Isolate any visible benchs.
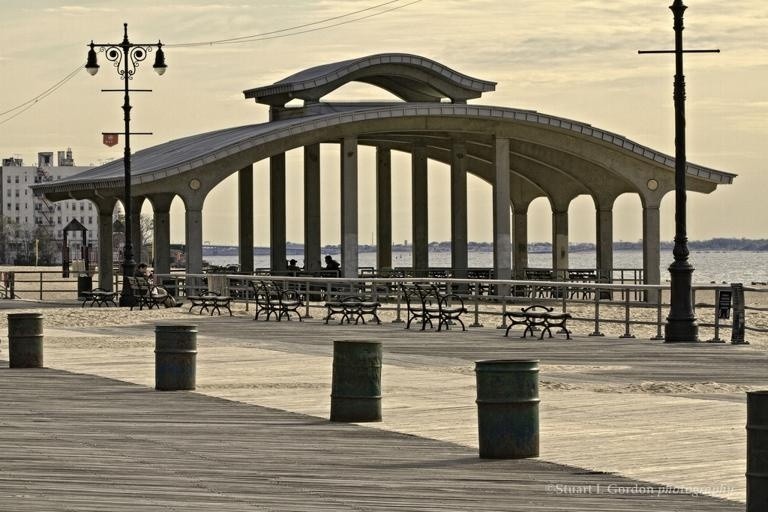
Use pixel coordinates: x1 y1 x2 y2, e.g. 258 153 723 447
322 285 572 342
81 280 303 325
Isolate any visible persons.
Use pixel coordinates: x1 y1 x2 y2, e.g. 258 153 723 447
287 259 301 276
323 255 341 277
134 262 183 308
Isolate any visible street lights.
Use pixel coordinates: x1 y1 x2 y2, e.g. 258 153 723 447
84 21 171 306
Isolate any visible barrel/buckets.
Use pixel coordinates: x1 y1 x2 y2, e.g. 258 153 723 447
474 358 540 460
330 340 383 422
78 277 92 299
746 390 768 512
7 313 43 367
154 325 198 391
